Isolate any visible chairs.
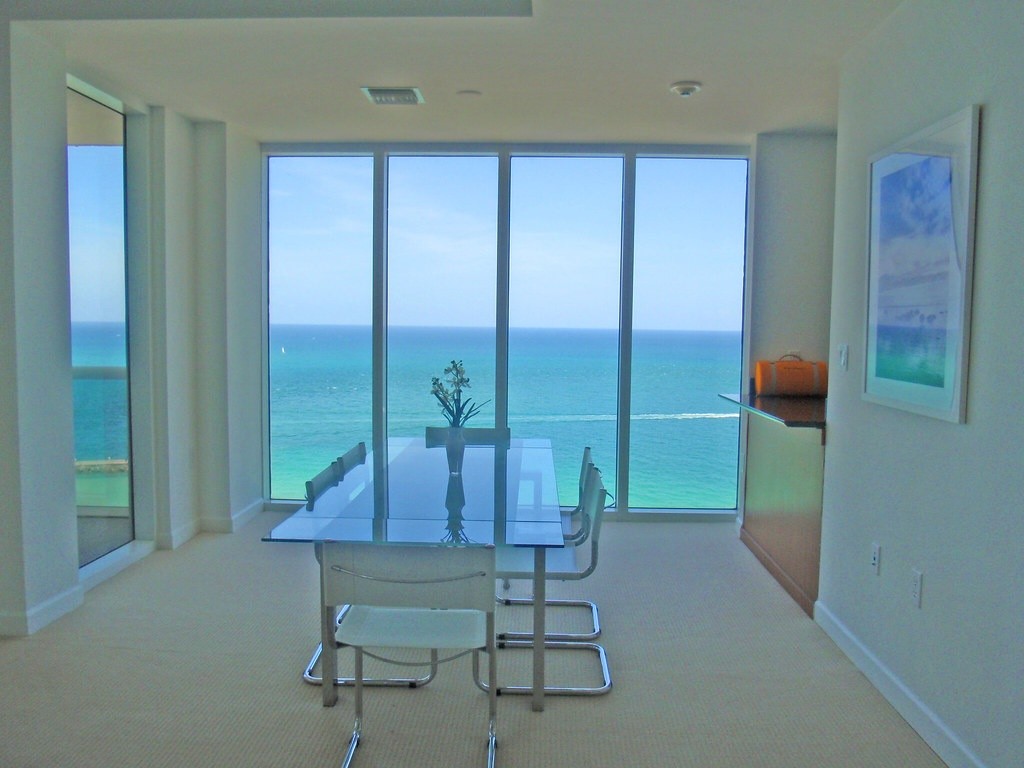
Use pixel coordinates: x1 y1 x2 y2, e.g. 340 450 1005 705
304 440 614 768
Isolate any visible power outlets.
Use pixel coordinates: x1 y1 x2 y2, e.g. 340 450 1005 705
907 568 922 609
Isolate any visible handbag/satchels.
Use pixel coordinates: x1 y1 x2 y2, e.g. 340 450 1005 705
755 354 828 396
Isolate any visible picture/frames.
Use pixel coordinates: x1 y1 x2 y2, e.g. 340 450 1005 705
861 104 983 426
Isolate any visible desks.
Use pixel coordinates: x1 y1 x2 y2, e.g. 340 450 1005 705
263 437 564 714
719 391 826 620
